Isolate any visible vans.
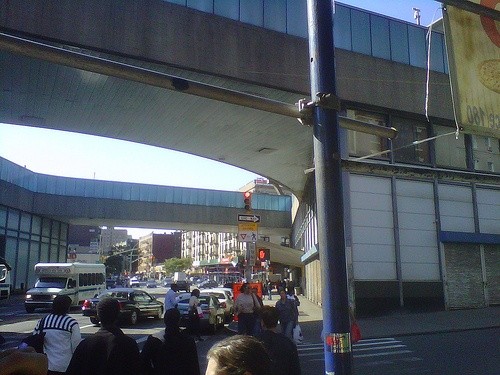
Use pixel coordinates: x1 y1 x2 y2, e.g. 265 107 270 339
25 263 107 313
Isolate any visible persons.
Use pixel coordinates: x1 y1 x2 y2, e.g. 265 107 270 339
276 286 300 338
221 277 294 300
188 289 204 340
258 305 300 375
165 284 180 310
152 308 201 375
234 283 263 335
206 335 274 375
33 295 81 375
66 297 147 375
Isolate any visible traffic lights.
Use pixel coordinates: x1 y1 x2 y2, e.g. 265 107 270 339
259 249 271 261
243 191 251 211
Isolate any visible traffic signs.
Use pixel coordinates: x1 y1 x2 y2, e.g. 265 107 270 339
237 214 261 224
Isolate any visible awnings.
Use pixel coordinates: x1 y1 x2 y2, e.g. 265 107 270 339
440 231 500 244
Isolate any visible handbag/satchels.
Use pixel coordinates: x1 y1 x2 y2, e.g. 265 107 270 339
293 324 304 344
189 304 201 322
18 315 47 353
251 293 262 315
164 303 186 325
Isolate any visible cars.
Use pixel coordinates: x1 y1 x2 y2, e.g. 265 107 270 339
83 288 164 326
107 271 234 329
